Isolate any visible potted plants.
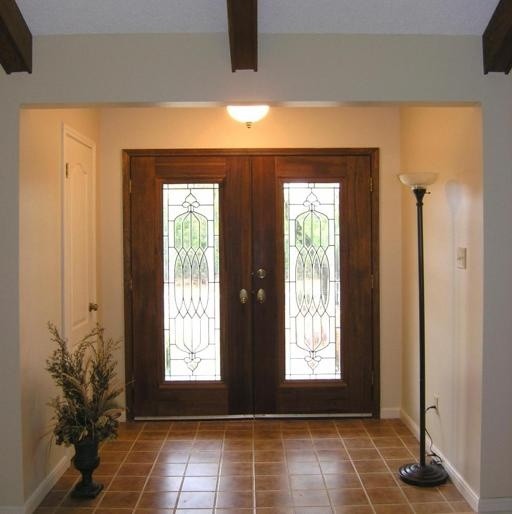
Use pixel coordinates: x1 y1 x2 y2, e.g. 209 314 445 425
46 321 131 498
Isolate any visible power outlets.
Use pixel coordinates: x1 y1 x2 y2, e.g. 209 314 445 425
433 393 439 416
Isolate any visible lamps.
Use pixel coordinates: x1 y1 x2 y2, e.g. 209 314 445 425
398 170 449 487
227 105 269 129
